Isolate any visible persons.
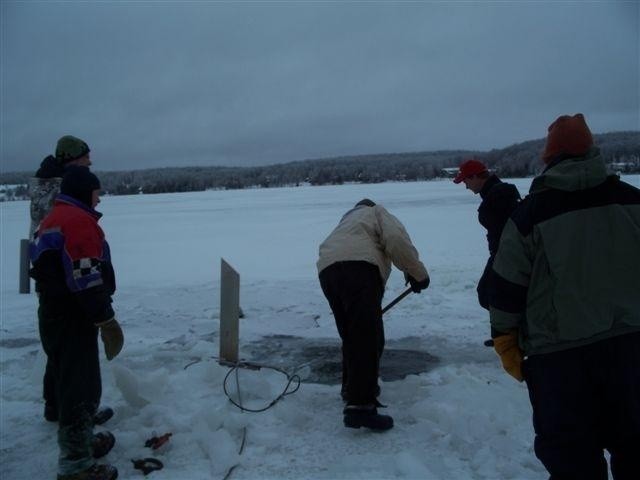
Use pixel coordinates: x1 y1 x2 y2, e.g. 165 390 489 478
26 165 126 480
317 198 431 434
29 134 113 426
483 111 639 480
451 154 523 349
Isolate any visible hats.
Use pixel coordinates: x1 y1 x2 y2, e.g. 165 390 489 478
453 160 488 184
55 135 90 163
542 114 593 163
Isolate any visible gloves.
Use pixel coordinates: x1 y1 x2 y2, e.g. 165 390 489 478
492 331 524 383
405 273 429 294
100 319 125 361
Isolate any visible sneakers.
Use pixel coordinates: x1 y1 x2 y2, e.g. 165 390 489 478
42 405 118 480
343 405 394 431
